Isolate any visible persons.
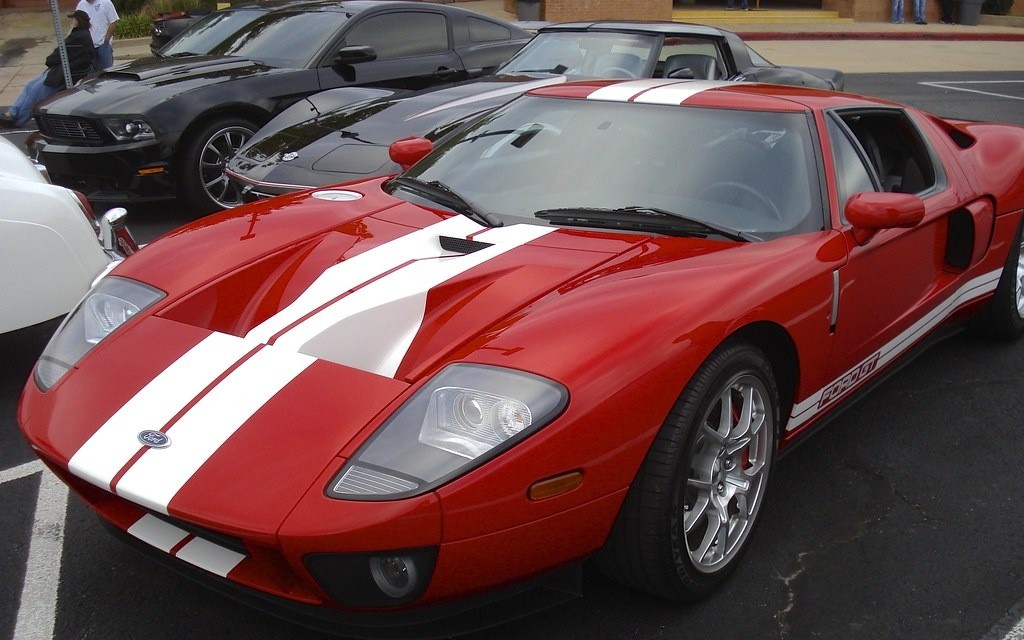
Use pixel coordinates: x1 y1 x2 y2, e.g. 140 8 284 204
891 0 927 24
0 10 96 129
76 0 120 76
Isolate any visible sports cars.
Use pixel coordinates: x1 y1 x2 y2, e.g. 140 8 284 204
226 17 845 219
17 76 1024 640
24 0 538 224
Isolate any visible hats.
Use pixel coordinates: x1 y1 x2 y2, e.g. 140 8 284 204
67 10 90 21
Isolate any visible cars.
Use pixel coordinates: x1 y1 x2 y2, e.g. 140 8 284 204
149 6 211 58
0 135 148 362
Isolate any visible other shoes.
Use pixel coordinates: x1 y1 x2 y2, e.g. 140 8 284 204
0 112 16 128
